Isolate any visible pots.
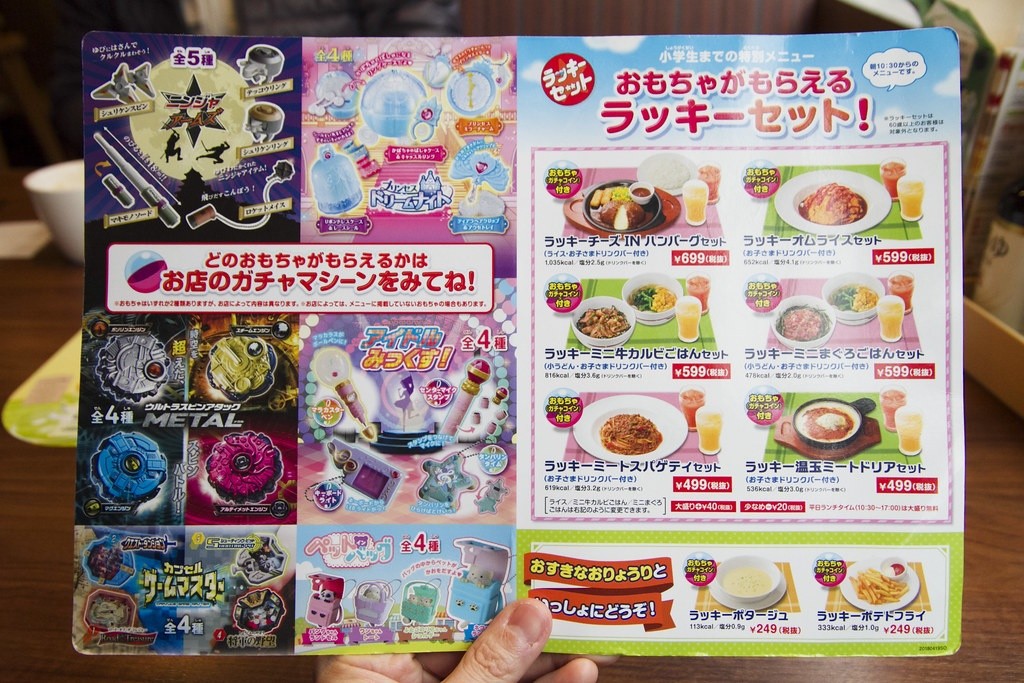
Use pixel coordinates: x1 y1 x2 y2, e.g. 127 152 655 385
792 397 876 449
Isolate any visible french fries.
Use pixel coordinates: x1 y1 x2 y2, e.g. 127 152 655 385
847 568 909 604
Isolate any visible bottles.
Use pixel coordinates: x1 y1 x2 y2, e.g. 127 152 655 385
970 178 1024 333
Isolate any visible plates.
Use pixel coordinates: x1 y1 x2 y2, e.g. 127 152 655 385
774 170 893 235
708 572 787 611
572 394 689 464
840 556 920 612
582 179 662 233
637 153 697 196
1 325 82 448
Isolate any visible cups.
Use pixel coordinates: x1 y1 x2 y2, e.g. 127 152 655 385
675 296 702 343
879 388 907 433
888 270 915 315
22 158 84 266
697 165 721 205
894 406 922 456
896 175 925 222
879 159 906 202
695 405 723 456
683 179 709 226
686 275 711 315
679 388 705 432
876 295 905 343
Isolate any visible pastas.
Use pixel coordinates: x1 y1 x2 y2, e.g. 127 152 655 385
602 414 657 454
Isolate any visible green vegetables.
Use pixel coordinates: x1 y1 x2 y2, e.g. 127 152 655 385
830 287 856 312
633 287 657 311
776 304 831 340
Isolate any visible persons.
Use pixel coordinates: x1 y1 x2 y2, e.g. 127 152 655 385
315 598 619 683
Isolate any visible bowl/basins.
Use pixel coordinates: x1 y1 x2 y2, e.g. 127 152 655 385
629 182 654 204
879 557 909 580
715 555 781 603
822 271 886 326
570 296 636 351
621 271 684 325
770 295 837 351
